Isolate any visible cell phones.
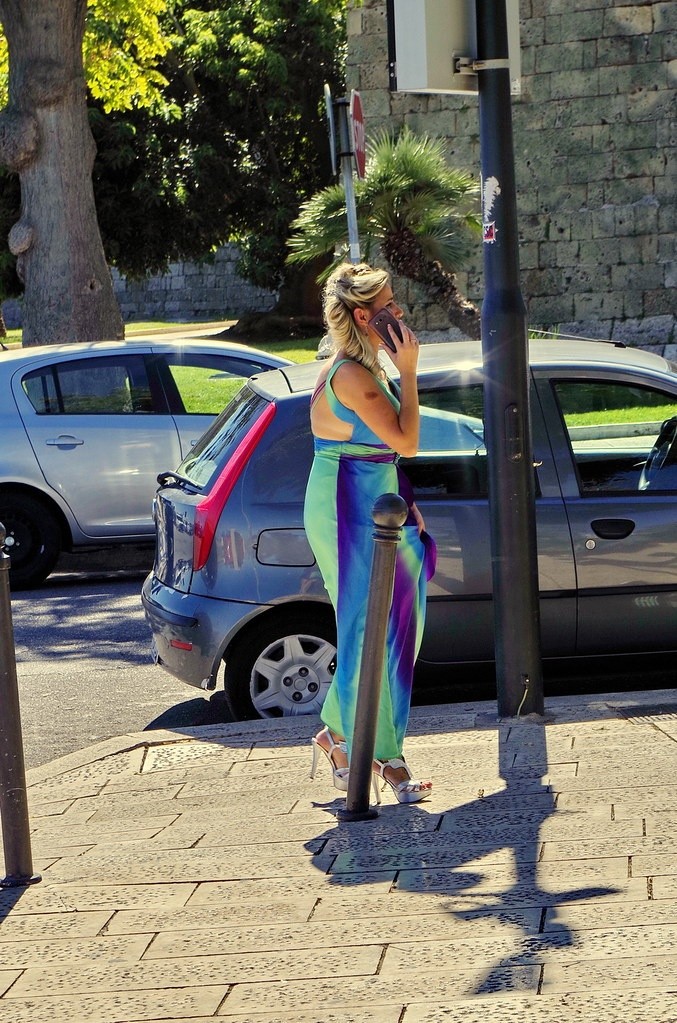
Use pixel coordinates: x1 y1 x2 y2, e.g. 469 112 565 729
368 308 404 353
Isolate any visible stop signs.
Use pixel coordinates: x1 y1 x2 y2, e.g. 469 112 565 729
346 89 367 183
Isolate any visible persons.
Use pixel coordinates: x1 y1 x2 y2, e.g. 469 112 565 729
304 265 437 806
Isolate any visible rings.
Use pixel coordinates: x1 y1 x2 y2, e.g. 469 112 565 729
410 339 417 342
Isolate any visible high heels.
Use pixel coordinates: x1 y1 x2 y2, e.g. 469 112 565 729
372 754 432 803
310 726 348 792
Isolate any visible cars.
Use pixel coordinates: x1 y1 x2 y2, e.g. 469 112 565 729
139 332 676 718
0 337 297 589
